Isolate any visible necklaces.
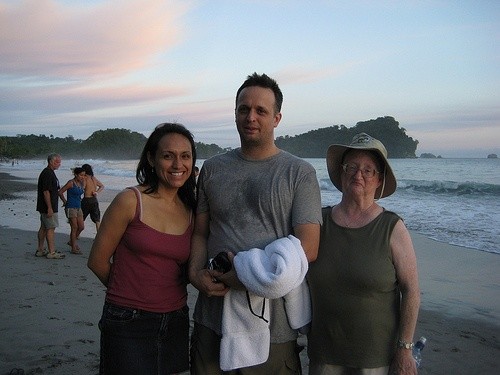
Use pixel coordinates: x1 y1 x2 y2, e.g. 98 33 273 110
340 202 375 229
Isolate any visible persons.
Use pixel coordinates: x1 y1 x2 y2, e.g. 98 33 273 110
305 132 421 375
59 167 84 254
194 166 199 178
35 153 67 258
81 164 104 231
188 72 322 375
87 122 195 375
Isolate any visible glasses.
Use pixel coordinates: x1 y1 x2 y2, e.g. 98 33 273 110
345 163 376 178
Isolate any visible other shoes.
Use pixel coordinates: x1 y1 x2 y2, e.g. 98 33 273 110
34 249 65 259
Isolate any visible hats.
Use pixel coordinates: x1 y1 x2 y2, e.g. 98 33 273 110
326 133 397 201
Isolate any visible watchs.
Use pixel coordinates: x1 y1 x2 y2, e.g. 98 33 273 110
398 339 414 350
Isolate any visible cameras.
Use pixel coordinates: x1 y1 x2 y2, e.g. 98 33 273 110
204 251 233 284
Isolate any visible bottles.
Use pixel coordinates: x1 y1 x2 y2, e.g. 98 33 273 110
388 336 427 375
